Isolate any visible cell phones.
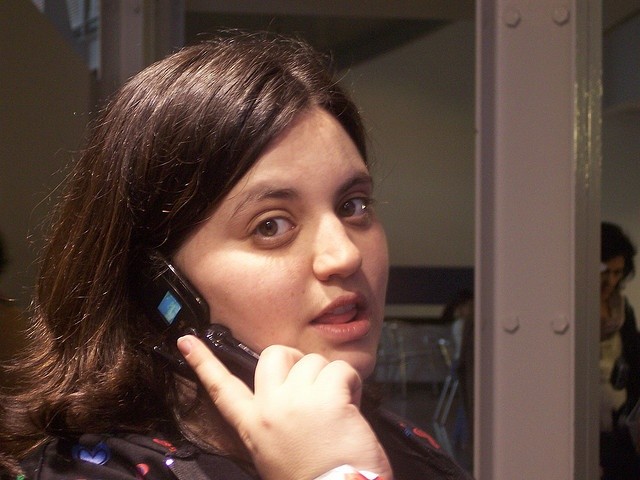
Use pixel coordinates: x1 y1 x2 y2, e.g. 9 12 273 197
132 253 260 394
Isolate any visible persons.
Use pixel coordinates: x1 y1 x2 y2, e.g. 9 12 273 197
0 28 477 480
599 221 640 480
